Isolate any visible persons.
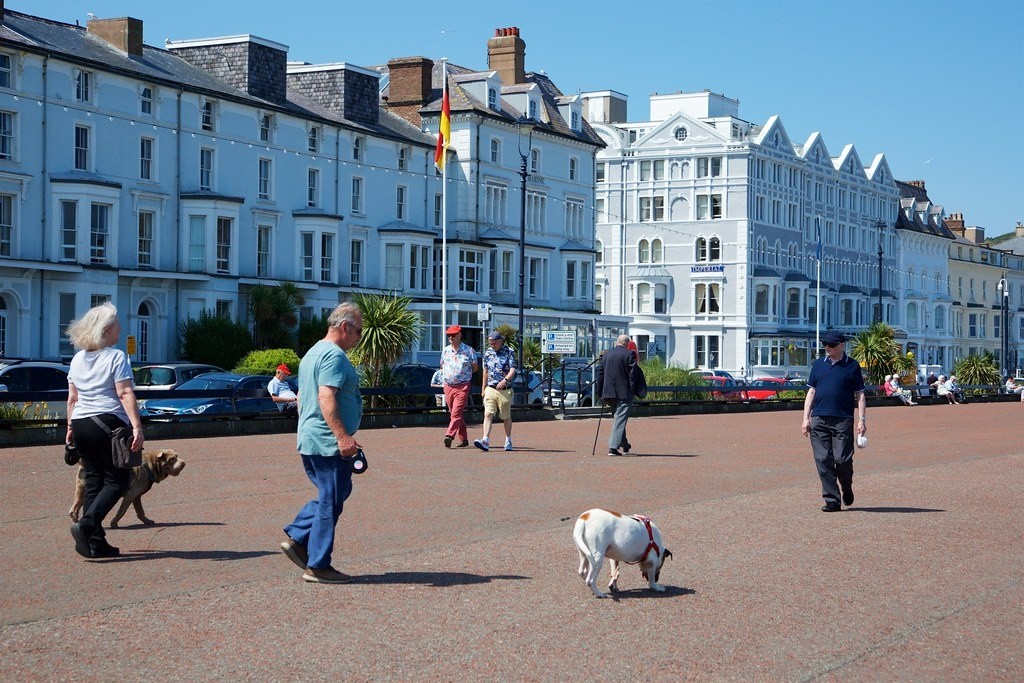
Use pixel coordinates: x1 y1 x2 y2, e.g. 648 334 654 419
66 302 144 558
431 365 443 406
927 372 938 398
267 365 297 413
945 376 968 404
916 374 922 396
884 373 918 405
890 374 912 405
801 330 867 511
930 375 959 405
281 302 364 584
441 325 477 447
1006 377 1024 402
596 334 637 456
474 331 517 451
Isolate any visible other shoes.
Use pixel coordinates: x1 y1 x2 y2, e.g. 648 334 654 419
623 444 631 452
821 501 841 512
474 439 489 451
608 448 622 456
456 440 468 447
842 486 854 506
505 441 512 450
280 538 309 569
303 564 352 583
69 522 92 557
89 538 120 557
444 437 452 447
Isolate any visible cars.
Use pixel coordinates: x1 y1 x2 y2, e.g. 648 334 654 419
0 357 70 429
138 369 299 424
133 363 229 403
509 360 600 409
356 362 436 408
689 370 809 404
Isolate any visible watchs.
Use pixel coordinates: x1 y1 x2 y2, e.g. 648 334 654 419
858 416 866 421
504 378 509 382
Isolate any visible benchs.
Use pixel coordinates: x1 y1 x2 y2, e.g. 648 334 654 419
628 385 1021 417
0 383 544 448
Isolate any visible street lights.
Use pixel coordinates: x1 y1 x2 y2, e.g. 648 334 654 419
513 109 541 370
997 277 1009 384
869 217 889 323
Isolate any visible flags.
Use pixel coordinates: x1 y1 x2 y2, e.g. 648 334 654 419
434 76 450 174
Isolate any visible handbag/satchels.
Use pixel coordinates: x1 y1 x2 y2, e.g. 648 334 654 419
111 425 143 467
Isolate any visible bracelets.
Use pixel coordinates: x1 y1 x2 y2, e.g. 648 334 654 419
133 428 143 432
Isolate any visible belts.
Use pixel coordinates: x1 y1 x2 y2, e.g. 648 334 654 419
489 386 512 390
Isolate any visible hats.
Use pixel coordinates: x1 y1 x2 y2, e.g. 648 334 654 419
820 330 845 344
488 331 506 343
893 374 900 378
885 375 891 381
277 365 291 374
951 376 956 379
446 325 461 335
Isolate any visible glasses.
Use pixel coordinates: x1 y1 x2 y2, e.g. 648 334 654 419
821 341 841 349
447 334 456 338
344 323 363 335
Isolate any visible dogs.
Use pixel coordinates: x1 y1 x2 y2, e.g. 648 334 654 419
67 447 188 531
570 505 676 600
